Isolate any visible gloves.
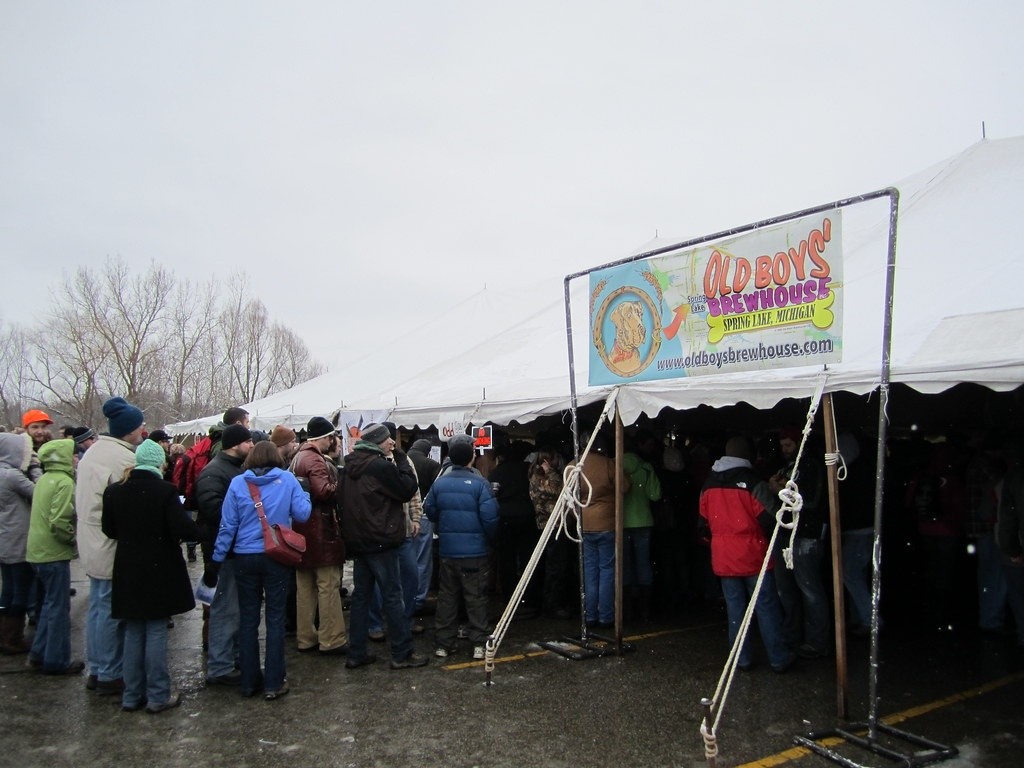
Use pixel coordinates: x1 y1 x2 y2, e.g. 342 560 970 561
203 560 221 588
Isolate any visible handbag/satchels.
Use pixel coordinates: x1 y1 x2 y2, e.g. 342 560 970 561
263 523 306 565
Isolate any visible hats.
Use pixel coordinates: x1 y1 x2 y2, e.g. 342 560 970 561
778 425 803 440
103 398 143 438
448 435 477 448
360 422 390 444
135 430 187 467
21 409 54 428
222 417 335 450
712 456 751 472
73 426 94 444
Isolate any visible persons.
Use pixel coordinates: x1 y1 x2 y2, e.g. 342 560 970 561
1 397 1023 712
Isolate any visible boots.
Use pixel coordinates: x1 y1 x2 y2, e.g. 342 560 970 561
0 607 32 654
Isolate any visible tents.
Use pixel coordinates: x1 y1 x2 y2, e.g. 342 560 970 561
162 134 1024 757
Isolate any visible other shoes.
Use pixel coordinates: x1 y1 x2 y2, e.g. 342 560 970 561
410 617 425 633
587 619 614 630
369 628 385 642
742 644 817 673
299 640 351 656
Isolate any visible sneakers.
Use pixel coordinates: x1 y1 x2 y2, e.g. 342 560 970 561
436 624 491 657
392 652 429 668
87 675 125 696
123 692 179 712
28 656 85 675
345 654 376 668
207 669 289 700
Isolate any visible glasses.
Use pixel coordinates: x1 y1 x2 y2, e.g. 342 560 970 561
328 435 333 441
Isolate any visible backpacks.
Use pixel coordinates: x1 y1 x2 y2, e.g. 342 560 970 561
174 433 213 499
